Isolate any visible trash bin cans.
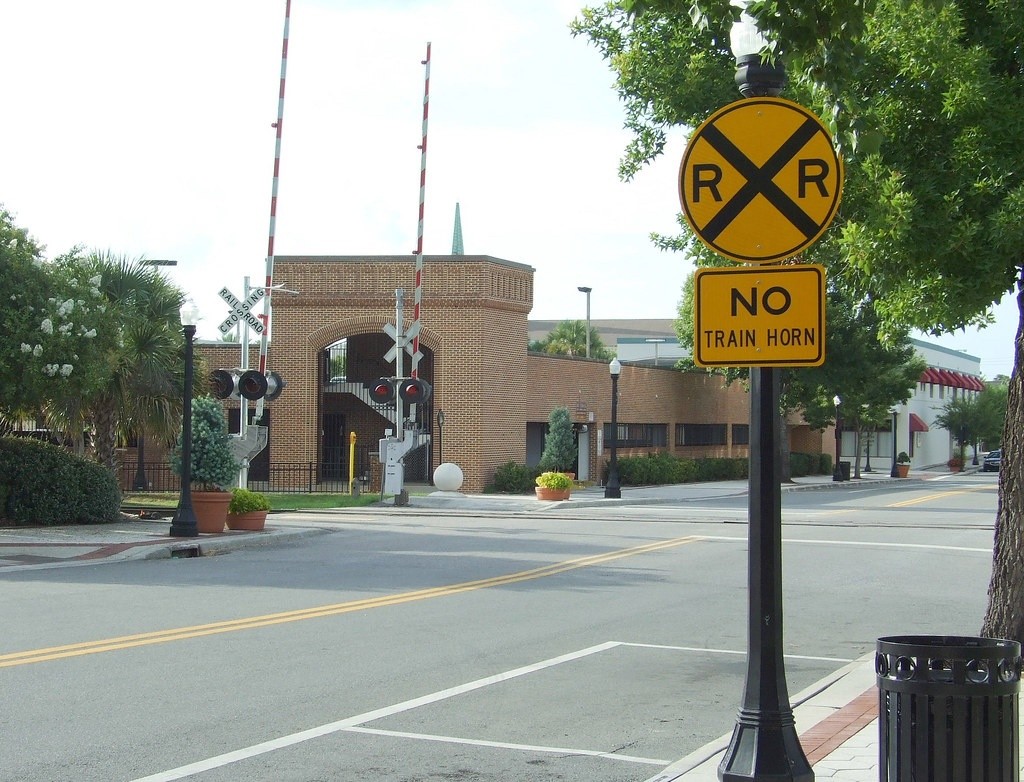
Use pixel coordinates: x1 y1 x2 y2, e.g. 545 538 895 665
874 635 1021 782
839 461 851 481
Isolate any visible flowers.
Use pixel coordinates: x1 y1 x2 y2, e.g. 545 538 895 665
947 458 961 467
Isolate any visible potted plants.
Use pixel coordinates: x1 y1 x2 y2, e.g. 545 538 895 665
173 393 249 533
540 405 576 500
896 452 911 478
535 472 574 501
225 487 270 530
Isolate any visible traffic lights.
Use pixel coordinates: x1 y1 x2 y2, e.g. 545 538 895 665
239 371 268 400
399 379 423 403
370 379 393 404
208 370 234 400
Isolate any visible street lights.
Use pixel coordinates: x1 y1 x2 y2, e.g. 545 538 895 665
168 299 199 537
833 395 844 482
578 287 592 357
891 402 900 478
604 357 621 498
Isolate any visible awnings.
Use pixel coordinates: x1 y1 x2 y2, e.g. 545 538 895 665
916 366 987 391
909 413 929 432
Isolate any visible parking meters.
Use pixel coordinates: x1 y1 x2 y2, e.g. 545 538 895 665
350 431 356 494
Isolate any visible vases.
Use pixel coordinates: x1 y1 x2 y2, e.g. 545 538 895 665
949 467 960 471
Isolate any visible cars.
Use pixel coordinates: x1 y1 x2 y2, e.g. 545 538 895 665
983 450 1000 472
